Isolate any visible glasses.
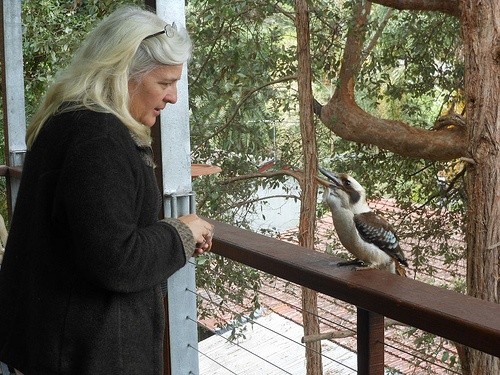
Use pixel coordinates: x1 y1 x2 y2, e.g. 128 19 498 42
143 21 177 41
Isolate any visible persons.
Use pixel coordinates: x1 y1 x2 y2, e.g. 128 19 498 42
1 5 214 375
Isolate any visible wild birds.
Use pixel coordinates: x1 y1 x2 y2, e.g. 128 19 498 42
313 167 409 279
